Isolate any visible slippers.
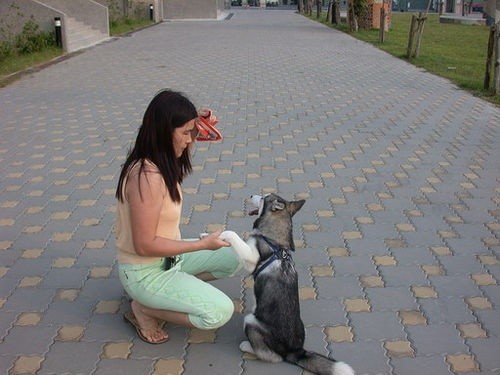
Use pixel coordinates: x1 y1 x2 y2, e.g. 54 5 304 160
124 309 169 343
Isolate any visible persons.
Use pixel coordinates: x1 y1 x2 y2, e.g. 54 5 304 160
114 89 247 344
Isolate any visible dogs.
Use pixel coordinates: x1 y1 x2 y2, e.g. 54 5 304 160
199 193 355 374
